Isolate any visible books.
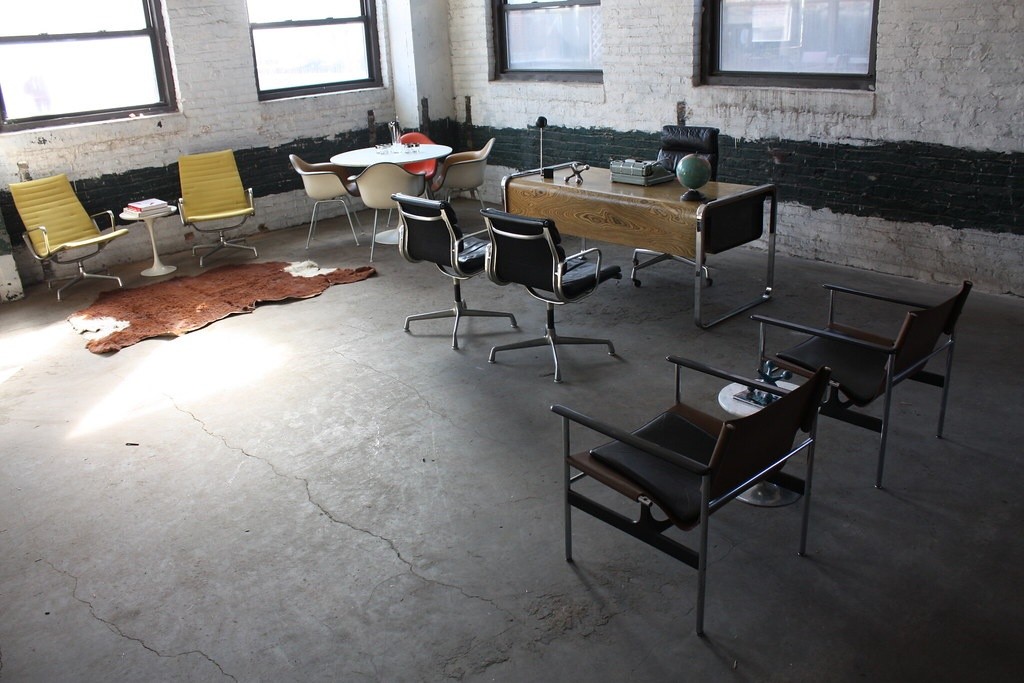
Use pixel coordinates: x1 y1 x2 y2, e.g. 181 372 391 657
123 207 171 217
127 197 168 212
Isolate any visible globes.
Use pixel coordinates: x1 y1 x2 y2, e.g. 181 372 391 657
676 151 712 201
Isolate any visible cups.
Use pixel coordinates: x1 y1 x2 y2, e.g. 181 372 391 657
404 142 419 153
375 143 391 154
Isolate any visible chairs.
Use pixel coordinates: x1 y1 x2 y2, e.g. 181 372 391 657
8 173 130 303
289 154 365 250
549 354 832 638
346 161 429 262
750 280 973 490
479 207 622 384
430 137 495 209
630 124 720 289
177 148 258 268
390 192 517 351
386 132 438 228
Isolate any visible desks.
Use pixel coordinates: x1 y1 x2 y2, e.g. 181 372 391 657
501 160 778 330
717 379 805 509
329 144 453 245
119 205 177 277
